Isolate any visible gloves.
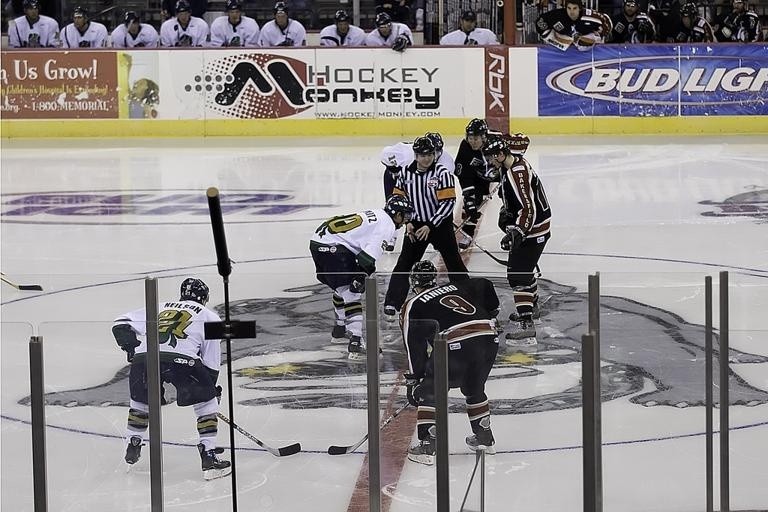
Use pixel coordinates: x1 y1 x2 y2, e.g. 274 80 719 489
403 373 426 407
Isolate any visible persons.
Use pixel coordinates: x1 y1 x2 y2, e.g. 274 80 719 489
0 0 501 55
309 193 414 356
534 0 767 54
112 278 231 471
381 119 552 341
399 260 498 456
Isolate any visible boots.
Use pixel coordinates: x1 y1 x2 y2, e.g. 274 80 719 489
197 443 230 471
332 305 540 355
408 423 437 456
466 414 495 446
125 437 145 464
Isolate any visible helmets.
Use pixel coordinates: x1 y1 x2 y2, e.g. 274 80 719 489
181 278 209 306
384 119 508 289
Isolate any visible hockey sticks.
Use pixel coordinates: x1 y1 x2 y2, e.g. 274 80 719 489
453 223 508 266
0 276 41 291
216 412 301 456
329 402 410 454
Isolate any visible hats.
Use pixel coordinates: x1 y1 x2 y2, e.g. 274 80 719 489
461 11 476 20
